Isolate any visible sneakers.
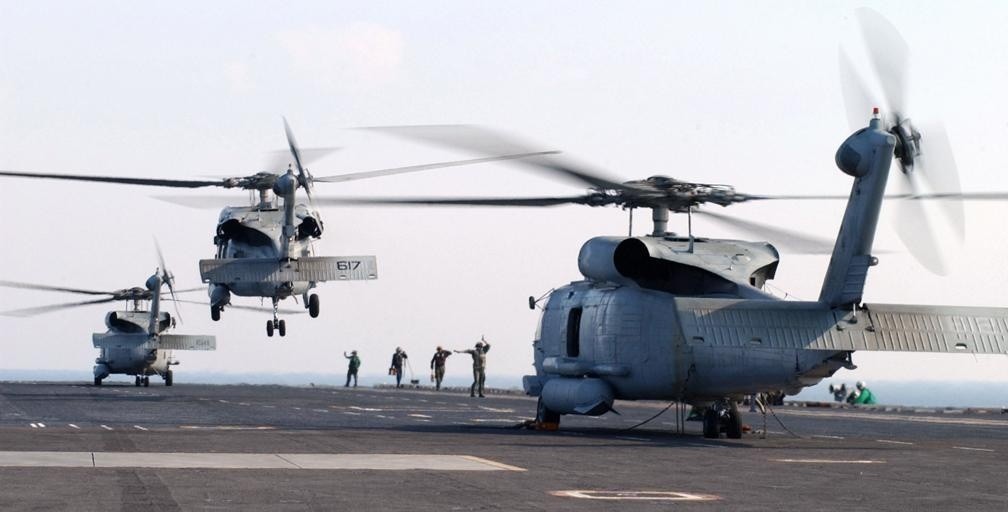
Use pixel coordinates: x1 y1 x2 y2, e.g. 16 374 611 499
470 394 476 397
478 394 486 398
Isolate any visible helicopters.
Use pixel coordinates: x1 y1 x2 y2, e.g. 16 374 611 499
0 118 574 337
367 5 1006 439
1 270 306 390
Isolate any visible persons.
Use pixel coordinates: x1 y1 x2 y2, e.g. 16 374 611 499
343 350 361 388
390 346 408 389
453 335 491 399
850 380 878 404
846 389 860 403
429 346 452 391
